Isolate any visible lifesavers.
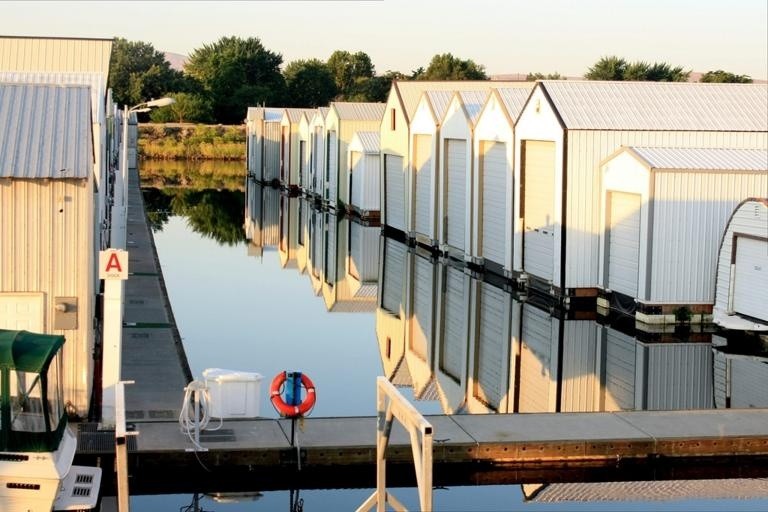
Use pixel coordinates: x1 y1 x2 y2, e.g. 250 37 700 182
269 370 316 415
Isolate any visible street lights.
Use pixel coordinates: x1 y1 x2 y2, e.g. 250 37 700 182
121 95 175 210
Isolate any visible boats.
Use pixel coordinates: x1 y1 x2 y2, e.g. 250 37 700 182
0 330 108 512
